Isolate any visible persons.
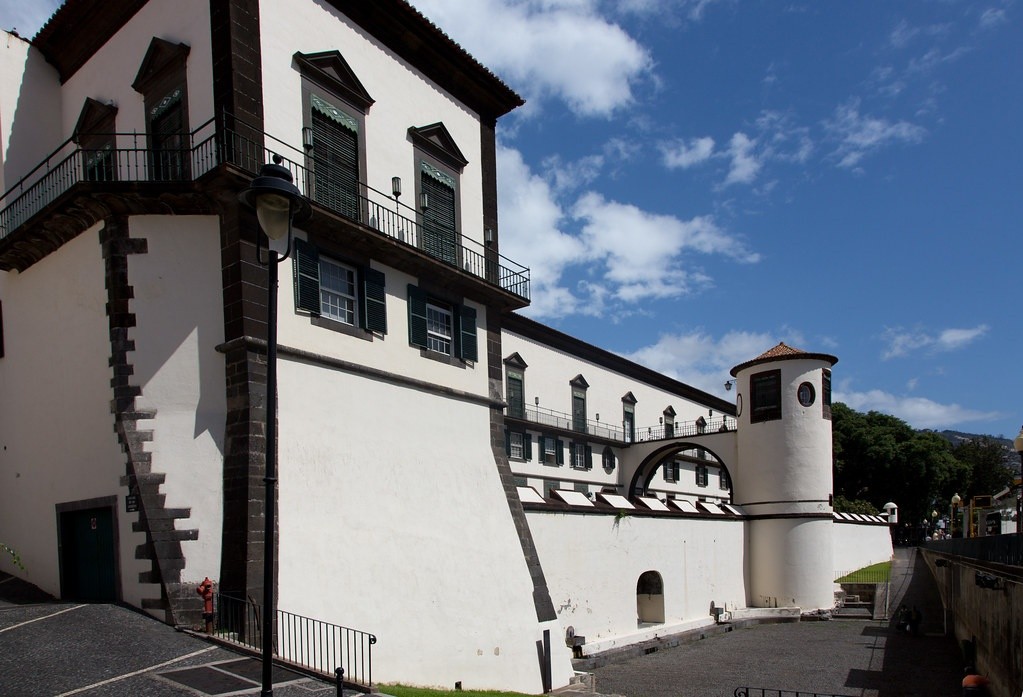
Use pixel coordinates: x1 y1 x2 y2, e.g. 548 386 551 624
899 605 922 636
926 523 963 542
962 666 991 688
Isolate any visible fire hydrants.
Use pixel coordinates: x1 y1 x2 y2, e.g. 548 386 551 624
196 576 213 634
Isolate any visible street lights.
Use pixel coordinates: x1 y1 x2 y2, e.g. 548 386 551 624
923 519 928 542
250 155 312 697
951 493 961 538
1013 425 1023 532
932 510 937 529
